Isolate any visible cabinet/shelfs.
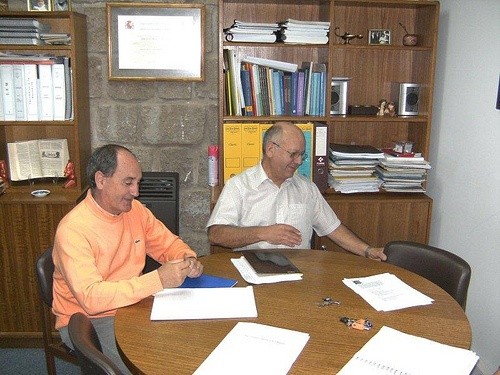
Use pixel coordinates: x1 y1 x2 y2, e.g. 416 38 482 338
0 11 92 348
211 0 440 259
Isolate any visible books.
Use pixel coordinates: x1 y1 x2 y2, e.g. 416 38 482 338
0 17 52 46
330 148 432 193
223 49 327 117
8 139 69 181
223 18 331 45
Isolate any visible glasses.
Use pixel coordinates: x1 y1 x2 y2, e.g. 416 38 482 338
268 140 308 161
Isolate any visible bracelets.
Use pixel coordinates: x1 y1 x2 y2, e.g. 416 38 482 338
365 247 371 258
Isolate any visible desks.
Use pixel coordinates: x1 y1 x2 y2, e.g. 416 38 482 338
111 249 474 375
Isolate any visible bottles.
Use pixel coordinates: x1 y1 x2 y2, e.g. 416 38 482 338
403 34 417 46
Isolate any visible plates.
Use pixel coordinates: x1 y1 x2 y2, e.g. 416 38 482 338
31 190 50 197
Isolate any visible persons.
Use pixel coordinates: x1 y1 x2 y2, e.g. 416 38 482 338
33 0 48 10
206 121 387 263
51 143 203 375
378 31 389 44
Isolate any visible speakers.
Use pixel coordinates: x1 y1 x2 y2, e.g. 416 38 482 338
330 80 348 115
390 82 420 116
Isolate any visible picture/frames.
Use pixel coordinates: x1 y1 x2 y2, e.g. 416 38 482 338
52 0 72 12
27 0 52 12
368 29 393 46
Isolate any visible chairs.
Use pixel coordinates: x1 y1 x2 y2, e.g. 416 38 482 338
380 241 471 312
36 246 124 375
